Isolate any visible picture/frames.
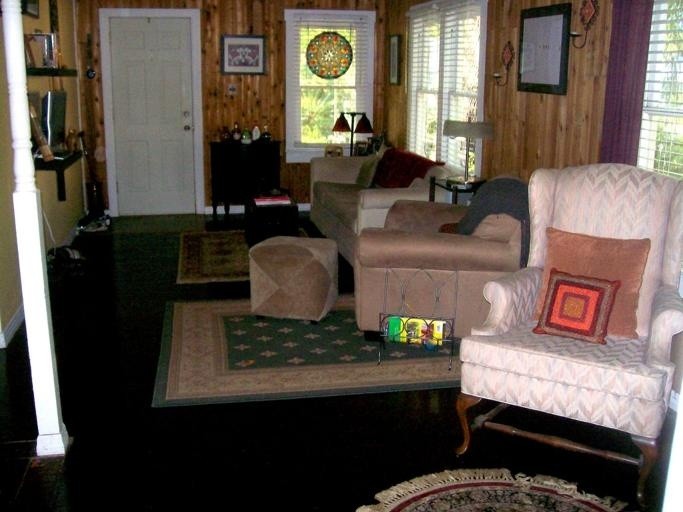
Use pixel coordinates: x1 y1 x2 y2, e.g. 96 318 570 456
21 0 39 18
220 33 267 75
516 2 572 95
388 33 402 86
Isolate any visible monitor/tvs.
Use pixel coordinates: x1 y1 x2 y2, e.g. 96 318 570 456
37 90 73 160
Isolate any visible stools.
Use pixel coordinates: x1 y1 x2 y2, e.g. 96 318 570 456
247 234 339 325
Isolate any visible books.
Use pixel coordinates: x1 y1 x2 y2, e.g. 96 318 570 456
28 30 61 69
254 196 291 205
387 317 445 347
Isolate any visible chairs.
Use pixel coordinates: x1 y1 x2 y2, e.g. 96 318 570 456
352 174 531 341
452 162 683 505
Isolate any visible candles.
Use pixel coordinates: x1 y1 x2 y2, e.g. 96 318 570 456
494 73 499 76
570 31 578 34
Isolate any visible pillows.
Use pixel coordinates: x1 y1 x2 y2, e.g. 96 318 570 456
527 226 650 338
438 221 460 235
533 266 622 347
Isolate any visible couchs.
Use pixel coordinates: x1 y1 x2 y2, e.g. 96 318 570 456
309 144 449 267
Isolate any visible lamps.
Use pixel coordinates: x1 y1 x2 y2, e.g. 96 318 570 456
442 116 494 184
332 111 374 156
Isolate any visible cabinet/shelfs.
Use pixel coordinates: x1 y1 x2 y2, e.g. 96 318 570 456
26 66 83 201
208 138 282 222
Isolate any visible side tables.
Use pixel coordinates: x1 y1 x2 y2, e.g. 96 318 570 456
429 174 489 205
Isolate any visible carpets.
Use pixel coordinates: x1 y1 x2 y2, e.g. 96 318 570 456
150 298 462 409
176 228 310 285
355 466 629 511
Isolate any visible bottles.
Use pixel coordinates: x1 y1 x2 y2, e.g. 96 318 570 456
241 128 251 139
261 126 272 142
232 122 241 140
251 126 261 141
